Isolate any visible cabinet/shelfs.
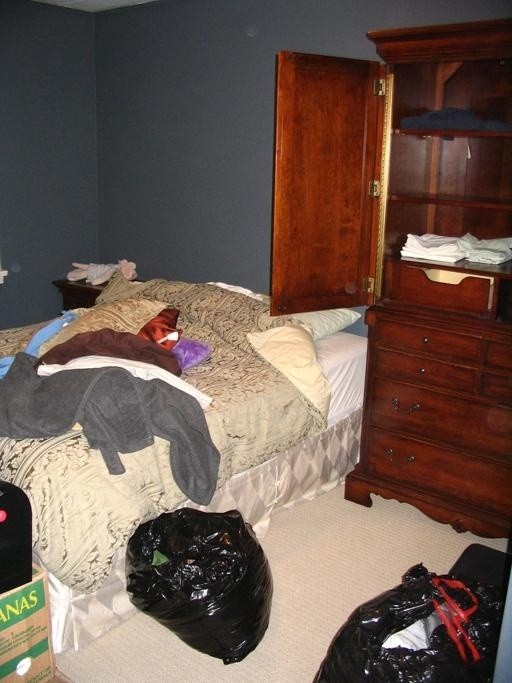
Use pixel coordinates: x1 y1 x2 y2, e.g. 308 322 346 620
268 16 512 543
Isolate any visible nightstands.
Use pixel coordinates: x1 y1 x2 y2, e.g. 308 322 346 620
50 277 147 311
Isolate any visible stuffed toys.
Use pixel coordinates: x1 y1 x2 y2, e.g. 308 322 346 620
65 258 140 286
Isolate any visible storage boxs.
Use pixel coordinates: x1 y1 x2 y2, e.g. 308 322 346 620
0 481 57 682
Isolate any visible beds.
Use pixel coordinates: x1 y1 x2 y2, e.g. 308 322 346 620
0 280 375 655
